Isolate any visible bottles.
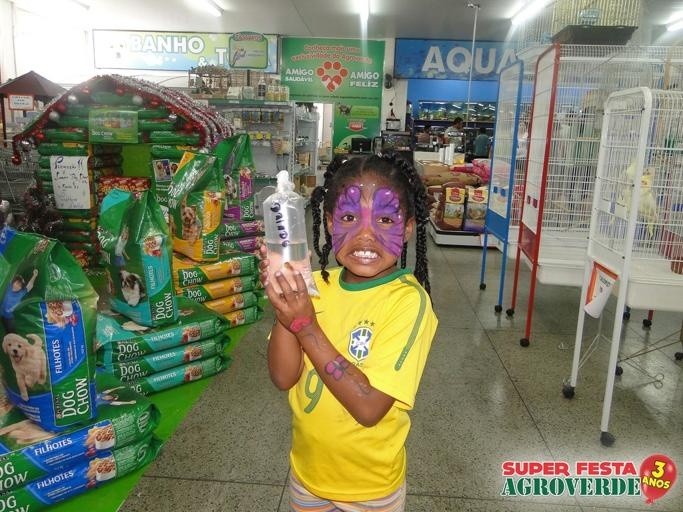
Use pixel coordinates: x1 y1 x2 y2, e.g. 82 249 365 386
257 69 268 101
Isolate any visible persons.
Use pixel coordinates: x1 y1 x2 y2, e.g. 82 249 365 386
95 385 137 409
417 118 600 201
114 224 130 270
0 269 38 334
257 148 439 512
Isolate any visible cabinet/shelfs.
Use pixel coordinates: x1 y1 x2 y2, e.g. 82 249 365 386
193 98 320 217
406 99 496 154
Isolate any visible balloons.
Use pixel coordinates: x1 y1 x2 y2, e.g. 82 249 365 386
639 453 678 505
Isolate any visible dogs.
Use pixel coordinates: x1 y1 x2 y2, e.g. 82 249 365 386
2 333 47 402
119 270 146 307
181 204 202 246
156 161 168 176
223 175 237 211
